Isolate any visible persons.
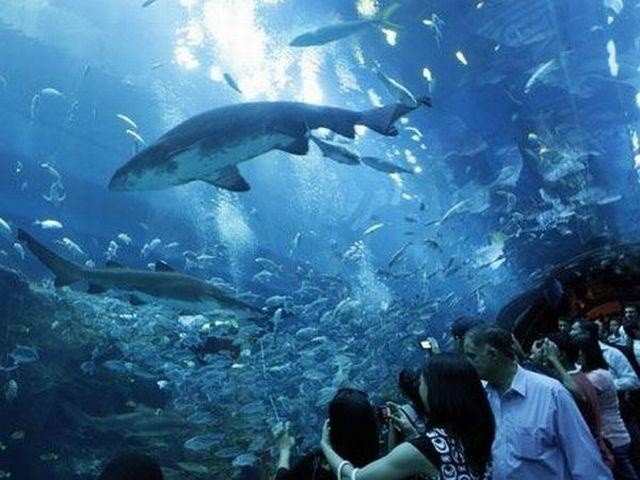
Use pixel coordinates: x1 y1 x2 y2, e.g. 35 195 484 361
272 247 639 480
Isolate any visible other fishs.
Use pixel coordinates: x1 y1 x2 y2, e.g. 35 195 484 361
0 0 638 479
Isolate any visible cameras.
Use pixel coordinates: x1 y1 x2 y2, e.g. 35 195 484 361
419 340 432 350
378 405 393 420
